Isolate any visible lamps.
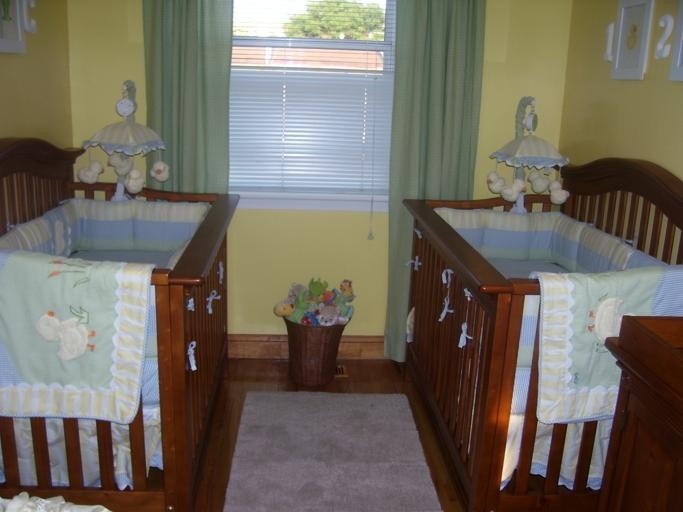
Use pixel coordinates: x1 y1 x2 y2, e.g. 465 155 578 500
486 94 570 216
77 78 170 203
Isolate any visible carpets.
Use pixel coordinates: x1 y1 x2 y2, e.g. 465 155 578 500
222 390 445 510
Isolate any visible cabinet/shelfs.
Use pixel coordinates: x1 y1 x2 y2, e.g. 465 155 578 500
591 313 682 512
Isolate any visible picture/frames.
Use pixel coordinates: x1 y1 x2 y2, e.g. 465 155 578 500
671 3 683 84
0 0 30 56
611 0 654 82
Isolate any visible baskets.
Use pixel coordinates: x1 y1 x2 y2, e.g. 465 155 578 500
283 315 346 387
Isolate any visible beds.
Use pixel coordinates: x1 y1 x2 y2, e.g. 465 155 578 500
400 156 681 510
0 135 242 512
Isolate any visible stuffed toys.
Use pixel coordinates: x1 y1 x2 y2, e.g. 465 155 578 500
482 96 571 210
271 275 356 327
78 79 170 201
